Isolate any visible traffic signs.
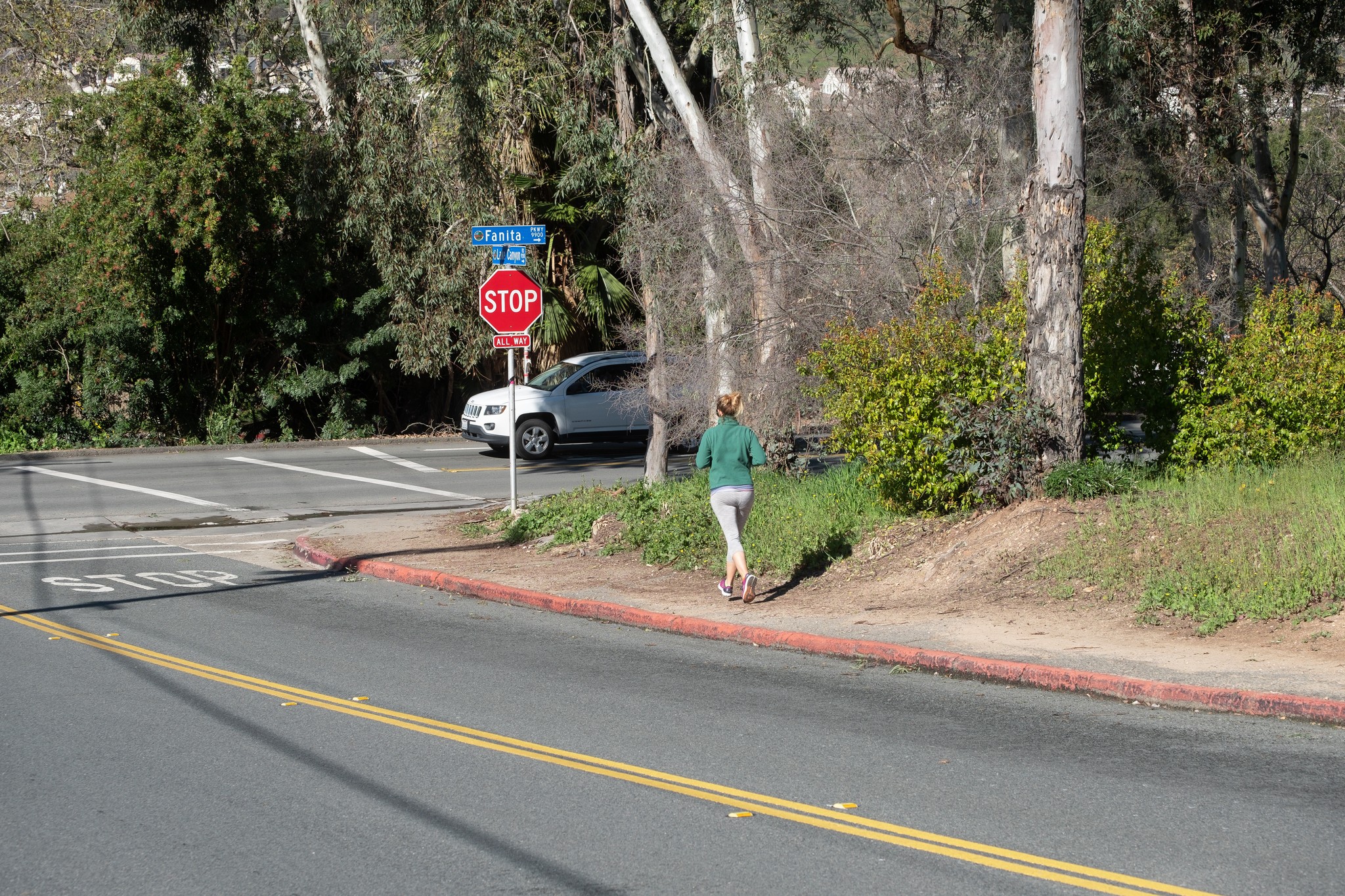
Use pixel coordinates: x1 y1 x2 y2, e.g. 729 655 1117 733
471 224 546 266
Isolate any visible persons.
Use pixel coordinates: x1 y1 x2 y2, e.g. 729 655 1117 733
694 391 769 602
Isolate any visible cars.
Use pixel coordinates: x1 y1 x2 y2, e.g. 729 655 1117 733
459 352 655 461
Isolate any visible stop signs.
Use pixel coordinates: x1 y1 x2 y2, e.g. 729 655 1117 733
478 268 544 349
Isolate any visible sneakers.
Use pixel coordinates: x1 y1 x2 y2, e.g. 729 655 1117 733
742 573 758 603
718 580 733 596
